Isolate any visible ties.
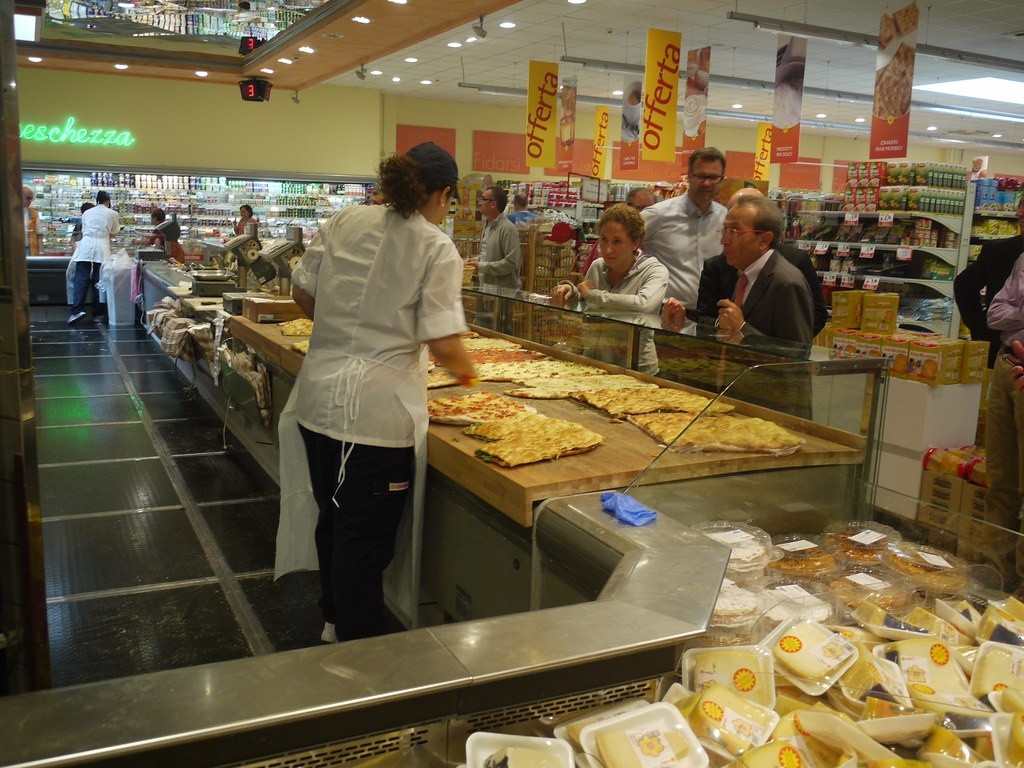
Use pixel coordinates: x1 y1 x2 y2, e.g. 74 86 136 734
715 273 748 392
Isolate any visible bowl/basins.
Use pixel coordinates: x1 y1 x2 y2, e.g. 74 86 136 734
466 514 1024 768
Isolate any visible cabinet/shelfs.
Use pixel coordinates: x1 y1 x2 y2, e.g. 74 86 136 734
795 179 1024 523
21 160 686 341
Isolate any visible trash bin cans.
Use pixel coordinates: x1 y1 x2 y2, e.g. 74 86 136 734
103 269 135 326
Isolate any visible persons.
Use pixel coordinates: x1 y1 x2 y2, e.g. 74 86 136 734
466 187 520 337
954 195 1023 588
71 203 96 255
22 187 45 257
583 147 731 319
132 208 185 264
67 191 120 325
293 143 476 644
550 205 669 380
233 205 258 274
662 188 828 420
508 193 540 228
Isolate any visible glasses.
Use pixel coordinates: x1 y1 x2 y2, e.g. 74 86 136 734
478 197 496 204
716 228 766 239
690 170 725 184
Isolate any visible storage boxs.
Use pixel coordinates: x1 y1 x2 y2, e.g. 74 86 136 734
243 296 313 322
918 469 1005 576
223 292 273 315
845 159 1024 257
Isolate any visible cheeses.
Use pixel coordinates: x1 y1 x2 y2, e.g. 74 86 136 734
483 597 1024 768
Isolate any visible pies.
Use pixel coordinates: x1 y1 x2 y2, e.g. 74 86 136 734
705 530 965 645
541 315 812 406
279 318 314 354
428 332 806 467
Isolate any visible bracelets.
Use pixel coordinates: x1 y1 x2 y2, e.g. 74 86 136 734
39 252 43 254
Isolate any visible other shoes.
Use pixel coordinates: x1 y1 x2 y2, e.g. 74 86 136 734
320 621 339 642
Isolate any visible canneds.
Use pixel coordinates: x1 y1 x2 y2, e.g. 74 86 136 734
900 220 955 249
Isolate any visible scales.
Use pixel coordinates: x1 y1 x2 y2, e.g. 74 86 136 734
192 223 263 296
136 214 180 260
222 227 306 316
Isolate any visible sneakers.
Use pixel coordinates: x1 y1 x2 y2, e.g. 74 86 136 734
66 311 86 325
94 314 108 324
